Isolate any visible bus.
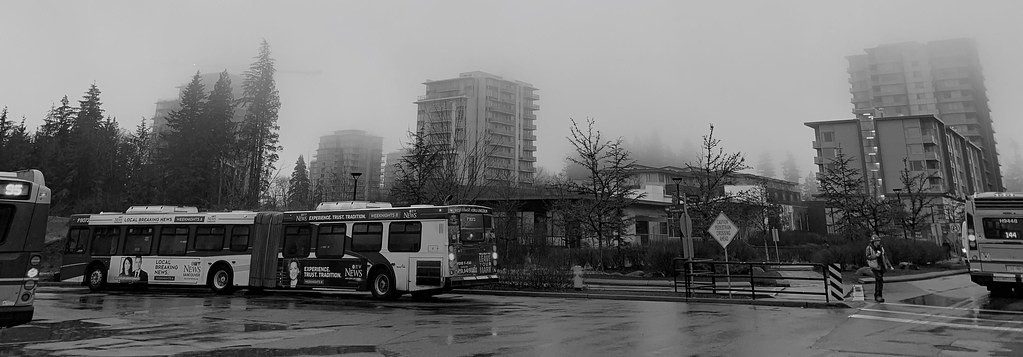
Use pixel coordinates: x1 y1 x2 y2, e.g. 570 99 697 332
59 201 498 301
961 191 1023 300
0 169 52 330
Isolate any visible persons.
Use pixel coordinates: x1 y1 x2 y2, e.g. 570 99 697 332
287 259 303 287
866 235 895 302
119 256 148 283
941 240 951 260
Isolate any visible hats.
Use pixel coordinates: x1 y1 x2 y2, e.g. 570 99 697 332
870 235 880 241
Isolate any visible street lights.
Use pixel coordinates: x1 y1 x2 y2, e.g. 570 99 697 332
670 175 685 205
350 172 364 200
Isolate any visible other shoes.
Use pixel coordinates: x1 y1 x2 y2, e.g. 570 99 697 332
875 296 884 302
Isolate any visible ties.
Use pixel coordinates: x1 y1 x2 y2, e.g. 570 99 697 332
136 272 138 278
